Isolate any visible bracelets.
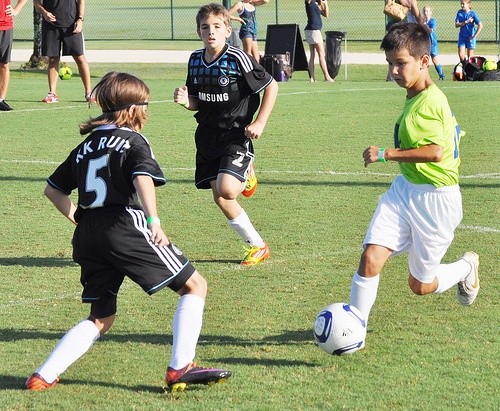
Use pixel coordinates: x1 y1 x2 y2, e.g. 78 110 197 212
78 16 85 21
378 146 388 163
179 100 187 106
237 17 240 21
147 216 160 225
464 20 468 24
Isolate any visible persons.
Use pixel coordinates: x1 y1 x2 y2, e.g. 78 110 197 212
26 71 232 392
228 0 269 64
33 0 96 104
386 0 447 82
304 0 334 83
455 0 483 62
0 0 28 111
350 22 480 326
173 2 279 266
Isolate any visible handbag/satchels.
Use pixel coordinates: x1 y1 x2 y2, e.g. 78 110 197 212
453 56 500 82
383 0 410 21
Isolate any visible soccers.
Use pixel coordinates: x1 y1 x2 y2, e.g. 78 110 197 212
313 302 366 355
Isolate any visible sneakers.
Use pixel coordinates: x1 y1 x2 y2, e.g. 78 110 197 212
24 372 60 390
240 240 270 265
164 361 233 394
241 162 258 197
456 251 481 306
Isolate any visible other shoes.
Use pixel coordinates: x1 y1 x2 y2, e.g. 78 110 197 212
85 91 97 103
0 100 14 111
41 93 59 103
